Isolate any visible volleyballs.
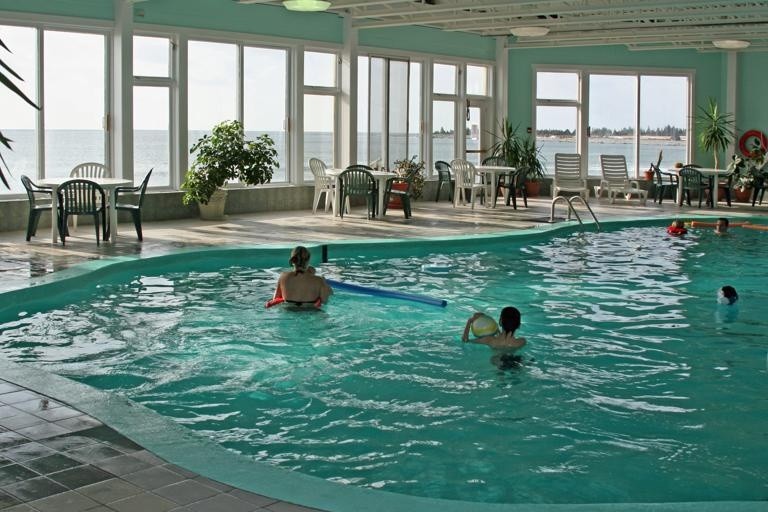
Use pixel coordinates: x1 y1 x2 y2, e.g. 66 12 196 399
718 285 738 305
472 315 498 338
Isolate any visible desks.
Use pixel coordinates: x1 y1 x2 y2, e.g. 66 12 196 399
455 165 515 208
326 168 397 219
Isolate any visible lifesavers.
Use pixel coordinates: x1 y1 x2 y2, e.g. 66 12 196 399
739 130 768 157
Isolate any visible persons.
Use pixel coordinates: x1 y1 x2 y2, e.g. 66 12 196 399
713 218 729 237
272 245 334 311
666 221 685 236
462 306 535 369
717 285 739 306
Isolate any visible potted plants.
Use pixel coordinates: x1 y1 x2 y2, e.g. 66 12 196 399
178 120 278 218
520 136 542 197
486 119 524 197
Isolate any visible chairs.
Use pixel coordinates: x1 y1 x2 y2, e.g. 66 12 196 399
481 157 505 205
551 152 590 206
450 159 487 210
383 165 422 220
434 160 453 204
20 162 153 245
493 167 530 211
593 154 648 207
338 170 377 221
309 158 350 217
347 165 371 169
650 163 735 206
